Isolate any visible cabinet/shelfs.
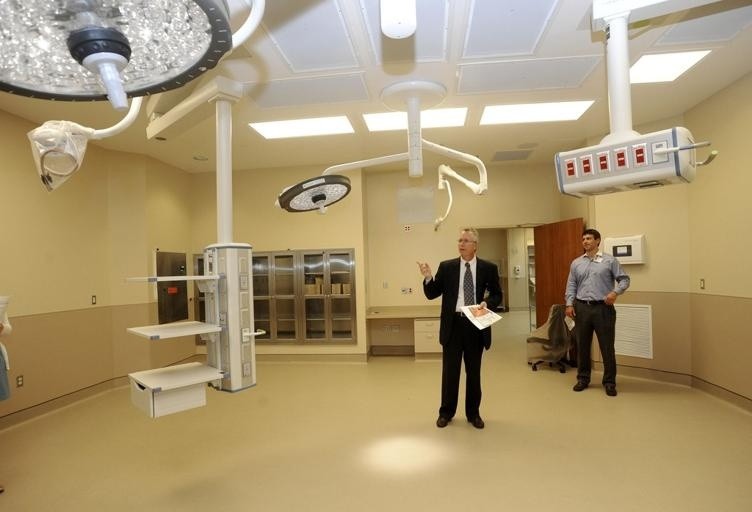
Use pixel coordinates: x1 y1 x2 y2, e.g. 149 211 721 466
122 274 228 419
249 248 358 346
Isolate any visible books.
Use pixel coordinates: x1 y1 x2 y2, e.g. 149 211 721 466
459 304 503 331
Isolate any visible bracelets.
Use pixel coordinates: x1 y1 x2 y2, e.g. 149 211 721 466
566 304 573 308
612 290 618 295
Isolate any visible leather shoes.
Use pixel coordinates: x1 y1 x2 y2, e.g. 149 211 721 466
606 384 617 396
573 381 588 391
436 416 448 427
465 412 485 429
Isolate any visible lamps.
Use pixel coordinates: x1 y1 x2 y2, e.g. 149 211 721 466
275 76 491 232
0 0 270 192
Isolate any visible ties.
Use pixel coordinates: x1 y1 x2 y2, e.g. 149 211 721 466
461 262 476 307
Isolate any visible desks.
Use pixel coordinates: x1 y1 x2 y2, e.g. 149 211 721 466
367 307 446 360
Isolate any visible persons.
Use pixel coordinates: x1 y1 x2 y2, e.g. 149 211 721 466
565 229 630 396
417 227 502 429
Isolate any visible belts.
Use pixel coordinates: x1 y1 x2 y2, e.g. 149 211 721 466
576 298 604 304
455 312 467 318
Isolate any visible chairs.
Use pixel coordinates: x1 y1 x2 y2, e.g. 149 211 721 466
525 303 578 373
